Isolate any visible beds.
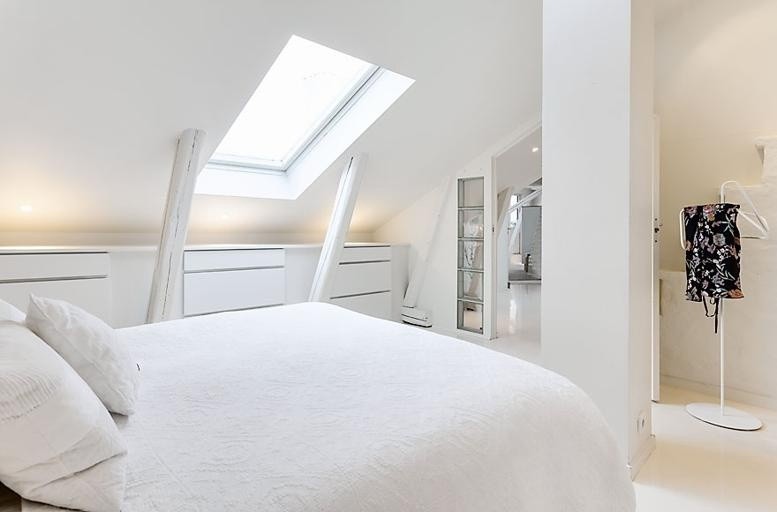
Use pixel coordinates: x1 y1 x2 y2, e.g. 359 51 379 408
0 302 638 512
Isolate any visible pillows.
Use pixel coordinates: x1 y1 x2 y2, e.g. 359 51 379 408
0 298 26 325
0 321 128 511
25 296 139 417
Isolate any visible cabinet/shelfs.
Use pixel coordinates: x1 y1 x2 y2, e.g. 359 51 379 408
183 245 286 316
0 247 111 325
329 243 391 320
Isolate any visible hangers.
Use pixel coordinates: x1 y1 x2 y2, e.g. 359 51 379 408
680 180 768 433
